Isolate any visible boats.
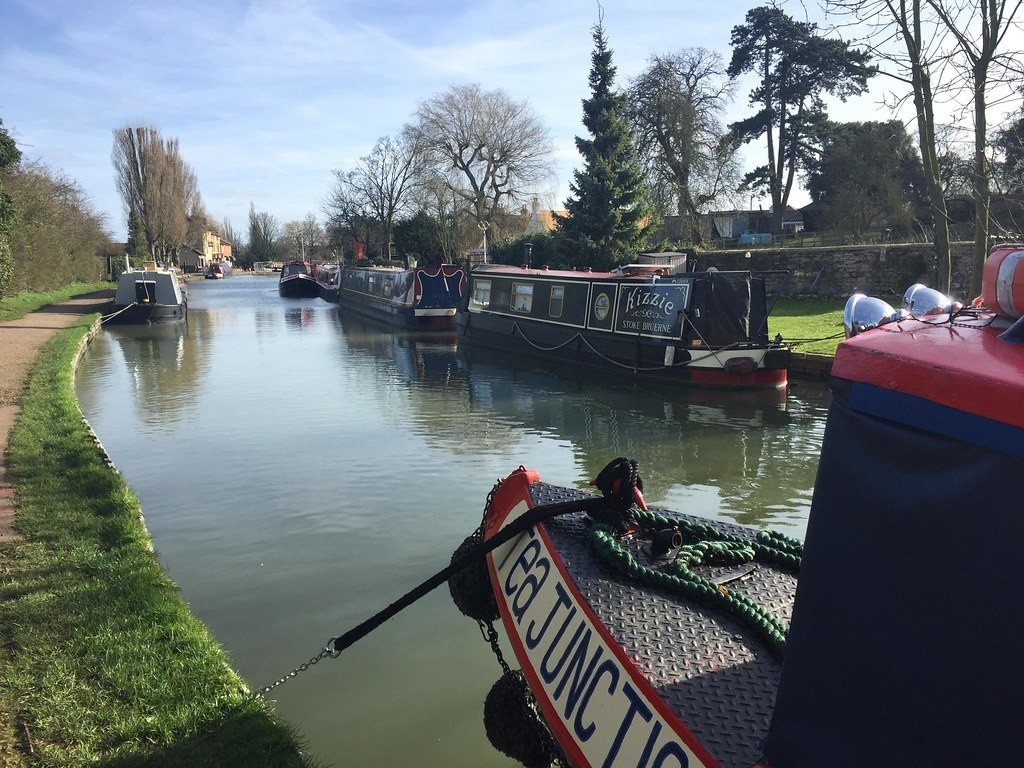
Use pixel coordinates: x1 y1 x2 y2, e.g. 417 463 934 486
338 254 468 317
480 237 1024 767
455 241 803 391
110 253 187 323
278 261 339 298
205 257 226 279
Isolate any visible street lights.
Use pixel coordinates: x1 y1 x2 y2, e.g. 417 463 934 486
477 221 490 264
